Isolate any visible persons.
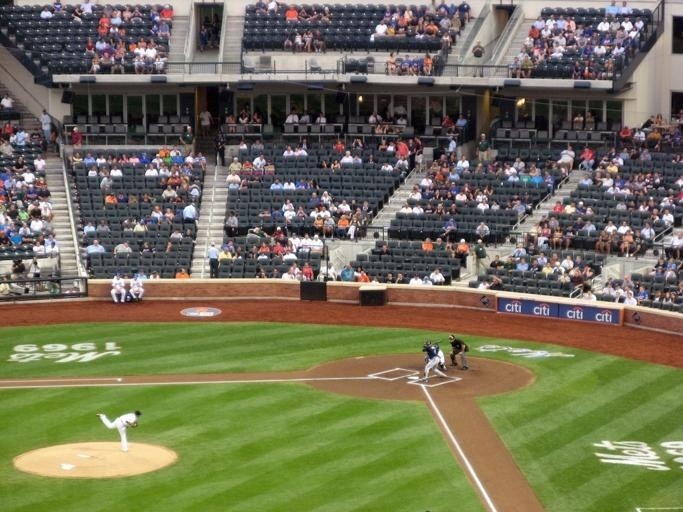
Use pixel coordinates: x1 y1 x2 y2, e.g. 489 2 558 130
199 14 221 51
1 94 60 293
422 342 446 371
40 0 173 74
472 40 485 75
421 110 683 305
65 107 213 303
446 334 471 370
207 110 423 280
254 0 473 74
421 339 446 380
94 409 142 454
510 0 648 80
